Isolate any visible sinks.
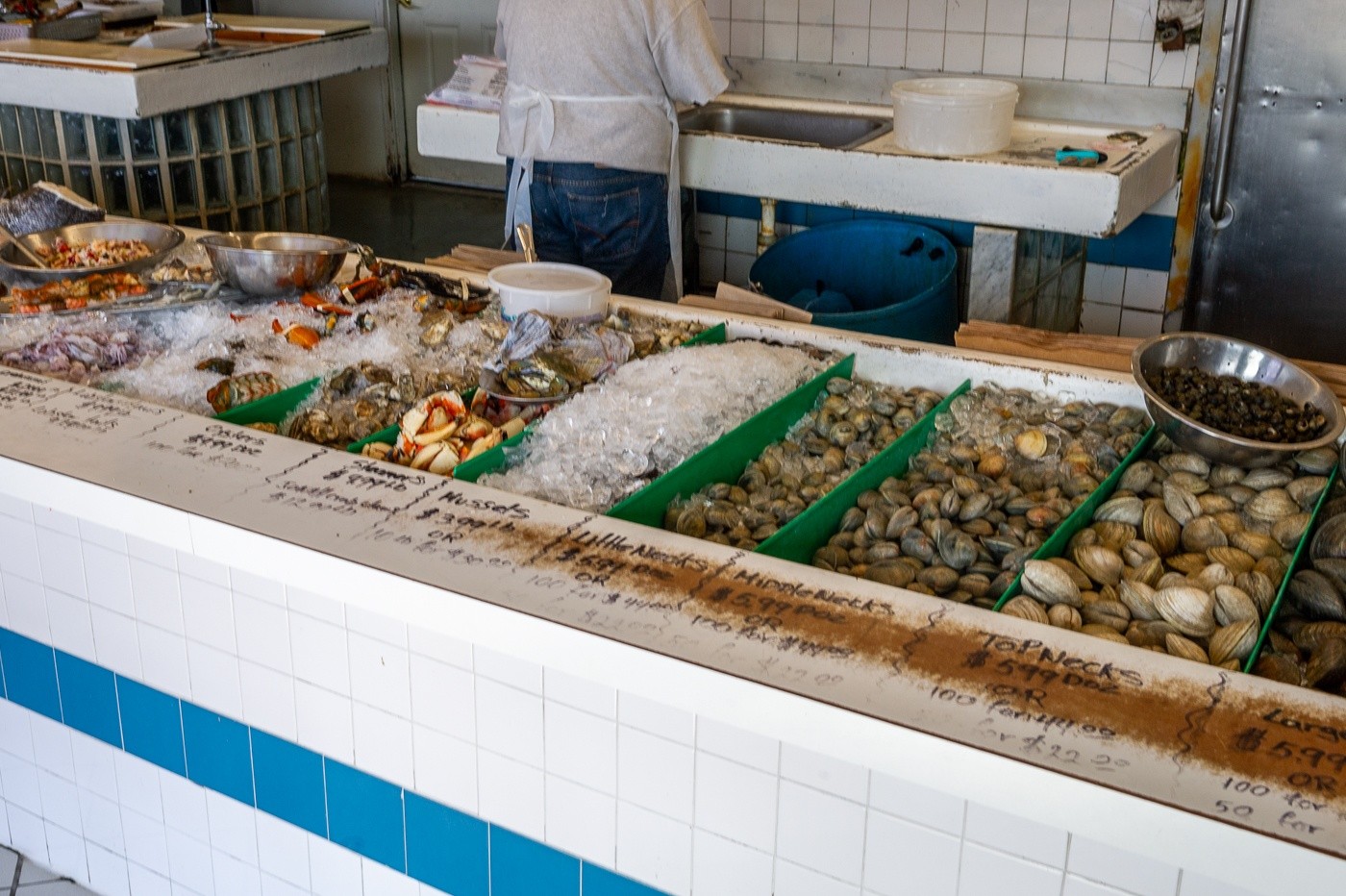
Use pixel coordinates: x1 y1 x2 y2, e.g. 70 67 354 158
674 100 894 152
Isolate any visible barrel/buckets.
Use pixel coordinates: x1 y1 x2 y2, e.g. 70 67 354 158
748 218 958 345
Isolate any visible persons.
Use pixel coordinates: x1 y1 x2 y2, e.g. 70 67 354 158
494 0 729 301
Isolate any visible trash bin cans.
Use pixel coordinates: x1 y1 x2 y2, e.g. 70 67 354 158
749 218 960 346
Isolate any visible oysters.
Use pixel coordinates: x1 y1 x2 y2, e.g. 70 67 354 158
245 314 508 449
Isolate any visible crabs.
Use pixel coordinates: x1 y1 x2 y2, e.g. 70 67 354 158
359 390 557 479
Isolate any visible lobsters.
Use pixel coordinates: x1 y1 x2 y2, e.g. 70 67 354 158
191 245 492 416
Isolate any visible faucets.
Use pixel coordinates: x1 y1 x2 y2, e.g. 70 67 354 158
203 0 228 49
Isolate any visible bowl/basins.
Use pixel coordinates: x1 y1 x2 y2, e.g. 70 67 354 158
0 219 185 286
887 76 1020 156
487 262 612 324
1132 331 1346 469
198 231 359 298
